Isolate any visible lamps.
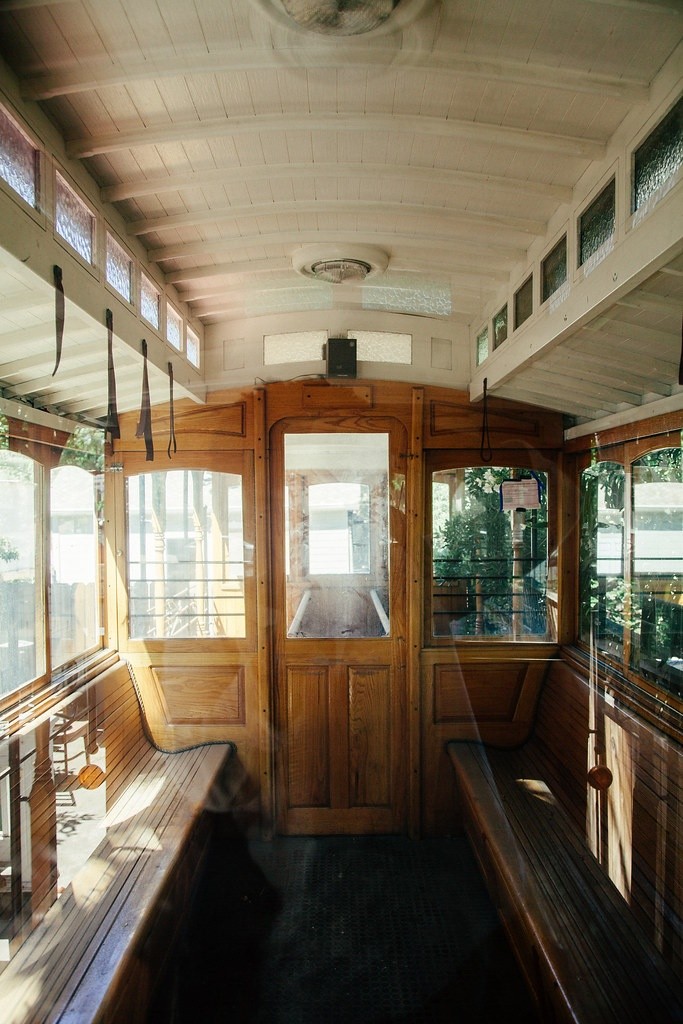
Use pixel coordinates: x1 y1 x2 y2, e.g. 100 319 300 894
252 0 434 41
291 245 389 286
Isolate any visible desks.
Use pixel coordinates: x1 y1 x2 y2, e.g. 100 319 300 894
53 720 90 774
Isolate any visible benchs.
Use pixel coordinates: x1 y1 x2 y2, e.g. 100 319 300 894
448 662 683 1024
0 660 235 1023
